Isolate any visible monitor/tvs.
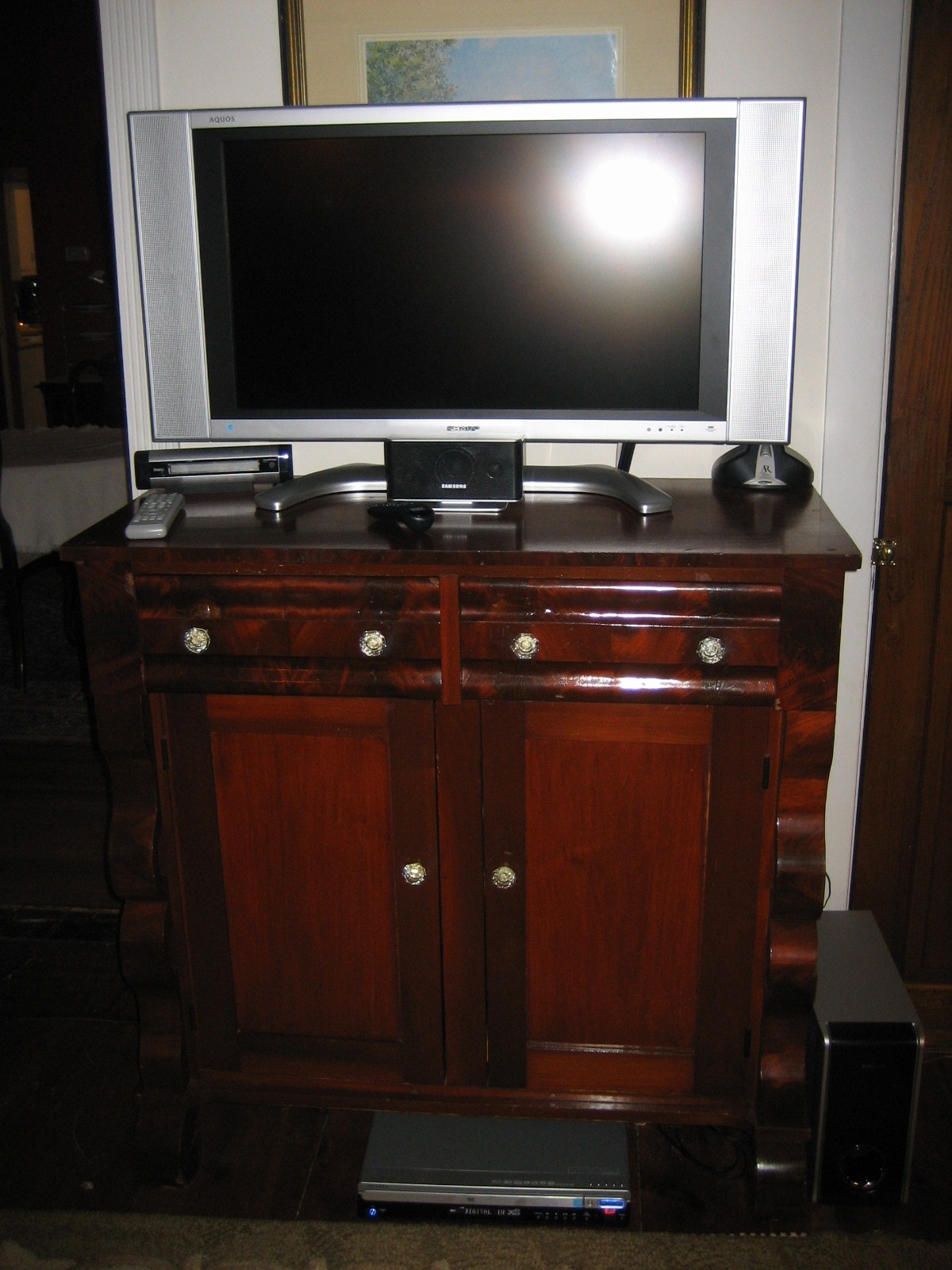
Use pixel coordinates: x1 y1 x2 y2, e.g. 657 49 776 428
126 94 807 442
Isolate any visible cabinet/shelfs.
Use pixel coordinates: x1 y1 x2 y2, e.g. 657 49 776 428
63 458 864 1183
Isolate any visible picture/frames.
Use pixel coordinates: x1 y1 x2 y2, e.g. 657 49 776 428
278 1 707 109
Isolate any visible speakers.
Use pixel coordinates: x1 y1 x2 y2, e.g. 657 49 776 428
806 910 928 1210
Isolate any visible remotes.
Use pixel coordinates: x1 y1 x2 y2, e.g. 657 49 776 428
125 491 185 540
366 500 437 534
